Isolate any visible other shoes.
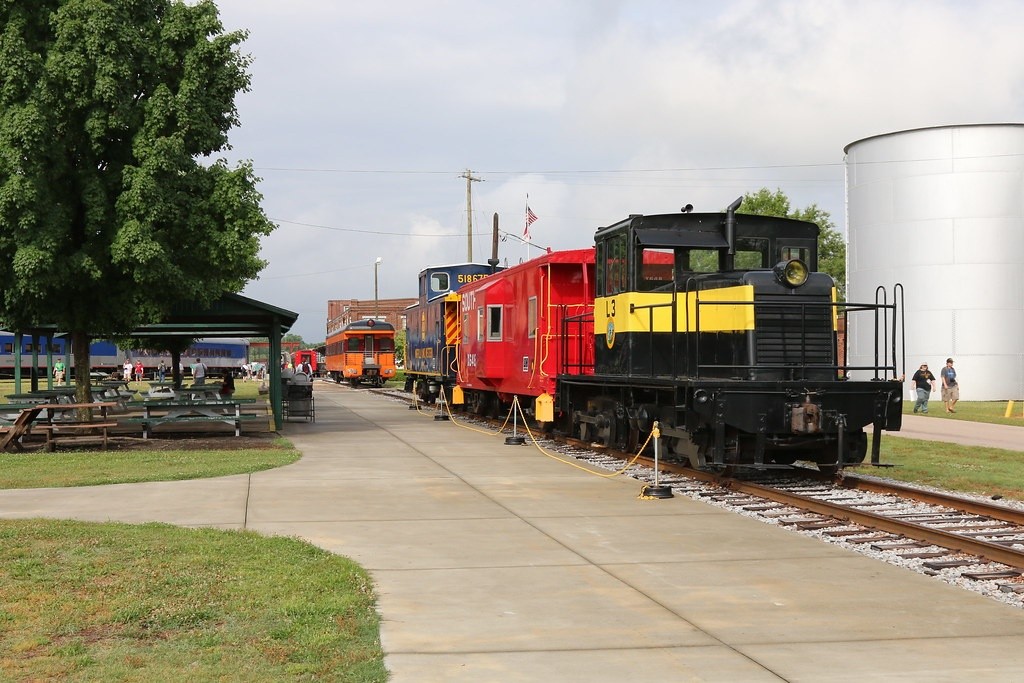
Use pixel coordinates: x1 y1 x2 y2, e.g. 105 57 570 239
948 407 955 413
914 407 917 413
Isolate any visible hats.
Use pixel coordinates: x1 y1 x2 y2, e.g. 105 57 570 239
947 358 954 363
302 358 307 362
58 358 61 360
921 362 927 366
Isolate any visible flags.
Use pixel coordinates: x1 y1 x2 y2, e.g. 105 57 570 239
524 201 537 237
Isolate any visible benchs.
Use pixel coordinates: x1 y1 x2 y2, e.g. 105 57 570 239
0 380 256 453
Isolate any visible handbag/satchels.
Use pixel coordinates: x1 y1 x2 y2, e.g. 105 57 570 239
202 364 207 377
241 366 245 372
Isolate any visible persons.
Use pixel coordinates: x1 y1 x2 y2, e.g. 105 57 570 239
53 358 65 386
216 369 235 395
170 358 184 374
296 358 313 376
941 358 959 413
911 362 936 414
158 360 167 382
241 363 249 383
193 358 207 384
123 358 133 381
134 361 143 382
284 361 292 369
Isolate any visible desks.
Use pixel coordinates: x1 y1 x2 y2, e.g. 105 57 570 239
188 383 222 400
0 404 37 453
126 398 256 440
4 392 57 426
92 384 121 398
103 380 130 400
35 390 77 404
148 382 177 398
35 401 117 451
53 384 107 402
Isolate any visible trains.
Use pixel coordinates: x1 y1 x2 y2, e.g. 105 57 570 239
288 349 318 379
0 330 252 381
402 194 907 479
325 319 398 388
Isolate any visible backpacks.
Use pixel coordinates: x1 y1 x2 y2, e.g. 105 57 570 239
160 363 165 373
302 362 311 375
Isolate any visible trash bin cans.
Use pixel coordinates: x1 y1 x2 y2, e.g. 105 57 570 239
286 372 313 416
281 368 293 399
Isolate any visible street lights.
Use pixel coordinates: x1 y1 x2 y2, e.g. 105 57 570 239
375 257 383 320
